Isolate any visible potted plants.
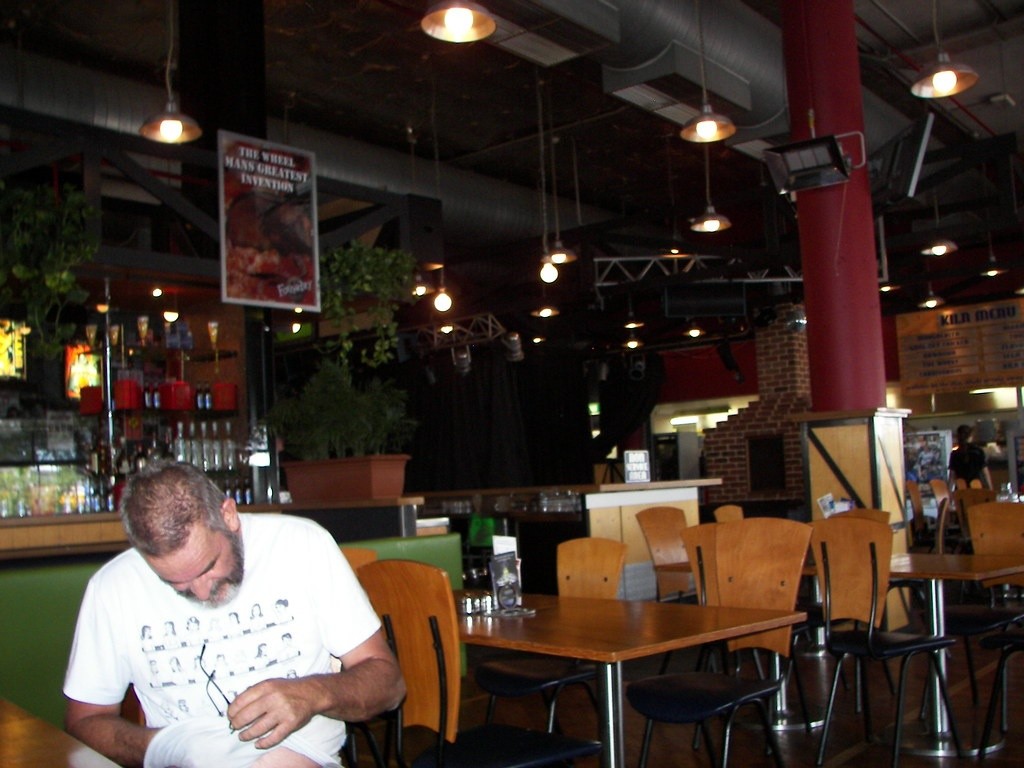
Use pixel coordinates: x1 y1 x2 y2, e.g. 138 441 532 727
251 243 418 502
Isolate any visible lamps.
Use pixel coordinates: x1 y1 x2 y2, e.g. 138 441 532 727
420 0 496 44
910 0 979 100
532 303 560 320
679 1 738 144
141 6 202 145
690 150 732 233
536 76 577 286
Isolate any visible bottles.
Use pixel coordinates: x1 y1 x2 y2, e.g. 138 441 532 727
212 422 223 472
152 381 160 409
1 475 119 516
116 427 173 474
194 381 204 412
175 422 186 462
234 480 244 504
244 479 253 504
204 381 212 411
189 423 203 472
224 423 238 472
200 423 214 472
143 380 152 409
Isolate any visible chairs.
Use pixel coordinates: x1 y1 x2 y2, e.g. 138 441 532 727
342 478 1024 768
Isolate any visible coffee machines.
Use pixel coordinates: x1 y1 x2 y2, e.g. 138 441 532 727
225 479 232 497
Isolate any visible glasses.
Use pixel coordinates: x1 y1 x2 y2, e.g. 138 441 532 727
198 644 234 717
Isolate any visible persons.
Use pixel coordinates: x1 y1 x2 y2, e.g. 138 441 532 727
915 435 942 483
946 424 992 494
64 460 407 768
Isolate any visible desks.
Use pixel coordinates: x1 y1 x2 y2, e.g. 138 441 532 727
462 594 808 768
802 550 1024 757
411 477 724 565
